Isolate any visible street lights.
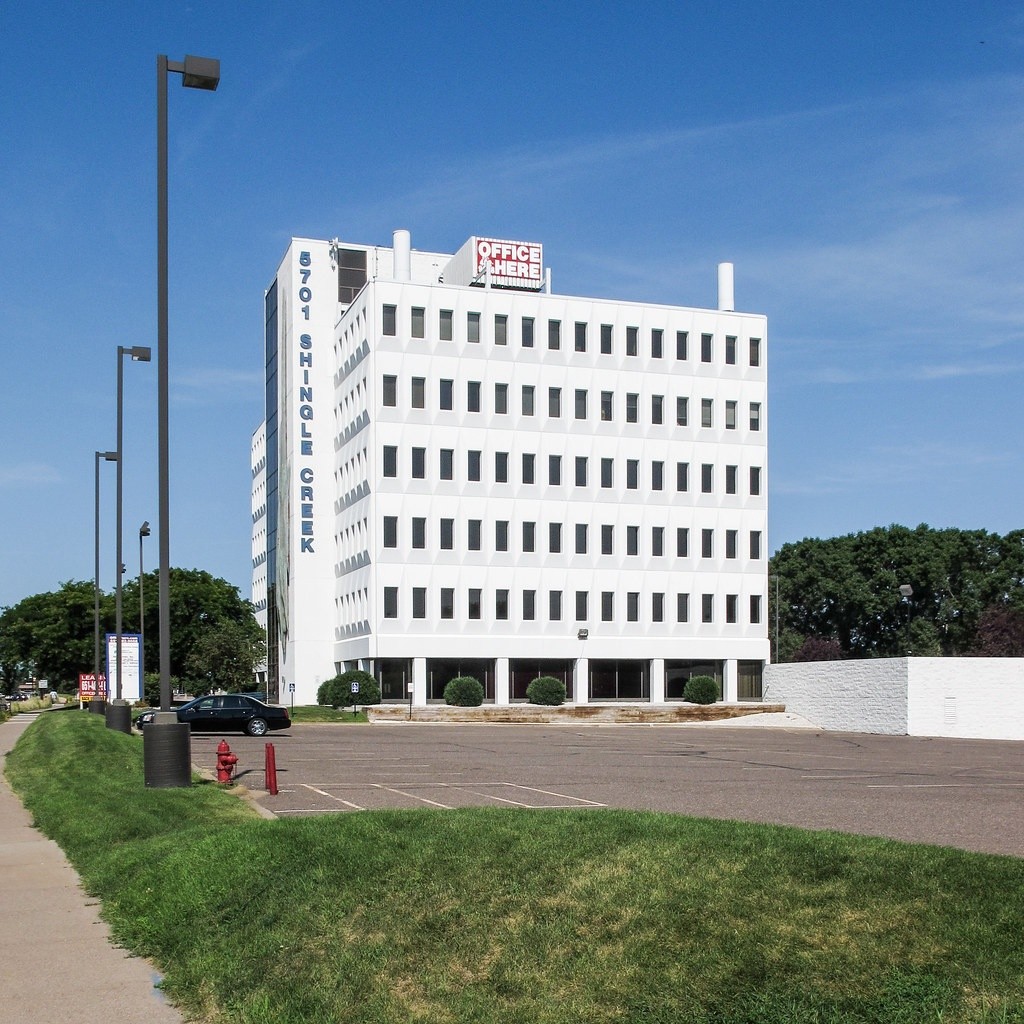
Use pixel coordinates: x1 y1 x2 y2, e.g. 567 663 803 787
103 345 151 732
899 584 915 656
136 518 151 715
139 50 227 787
88 449 118 715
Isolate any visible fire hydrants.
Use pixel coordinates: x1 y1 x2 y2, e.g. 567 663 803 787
215 739 238 785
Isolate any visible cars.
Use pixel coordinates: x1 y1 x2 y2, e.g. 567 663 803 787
137 693 293 738
6 693 22 702
